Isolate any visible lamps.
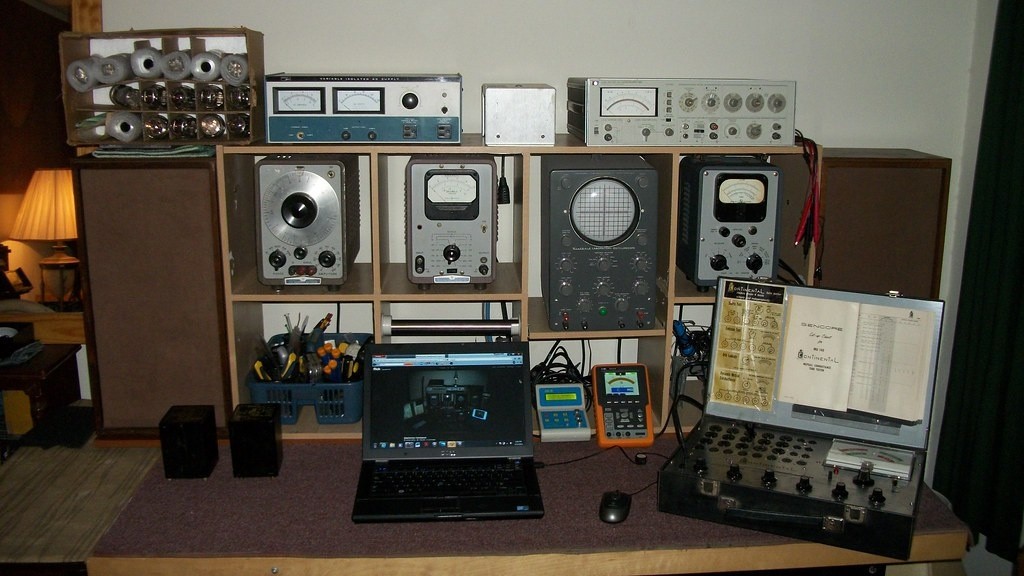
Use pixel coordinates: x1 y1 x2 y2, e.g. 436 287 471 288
8 169 83 310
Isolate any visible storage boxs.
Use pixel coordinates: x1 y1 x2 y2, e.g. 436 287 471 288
245 331 372 424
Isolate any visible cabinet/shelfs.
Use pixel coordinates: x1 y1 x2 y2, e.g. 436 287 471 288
216 145 822 440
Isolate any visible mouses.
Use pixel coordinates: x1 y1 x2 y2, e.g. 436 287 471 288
598 489 632 525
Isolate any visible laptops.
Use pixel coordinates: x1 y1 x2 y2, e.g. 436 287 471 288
352 341 544 524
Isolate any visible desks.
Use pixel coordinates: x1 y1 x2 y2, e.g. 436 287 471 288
0 396 967 576
0 300 86 426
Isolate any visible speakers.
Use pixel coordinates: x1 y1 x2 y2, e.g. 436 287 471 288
158 404 219 481
229 404 284 479
